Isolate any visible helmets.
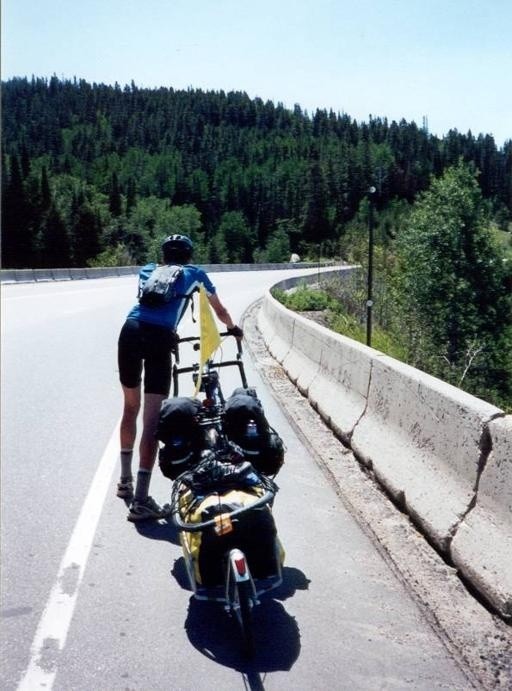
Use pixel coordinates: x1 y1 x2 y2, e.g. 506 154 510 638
162 235 194 253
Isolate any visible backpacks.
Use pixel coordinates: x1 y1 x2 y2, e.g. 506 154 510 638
138 264 186 304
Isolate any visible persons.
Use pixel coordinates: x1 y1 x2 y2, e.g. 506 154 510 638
116 233 244 521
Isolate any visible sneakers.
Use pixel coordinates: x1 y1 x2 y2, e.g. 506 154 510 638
117 480 134 497
127 496 170 521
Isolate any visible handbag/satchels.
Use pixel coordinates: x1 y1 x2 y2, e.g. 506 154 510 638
223 387 284 472
180 483 284 586
156 397 202 480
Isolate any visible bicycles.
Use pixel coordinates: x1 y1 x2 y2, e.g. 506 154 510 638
167 331 284 663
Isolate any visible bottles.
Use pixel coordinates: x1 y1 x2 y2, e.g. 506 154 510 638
247 420 258 438
